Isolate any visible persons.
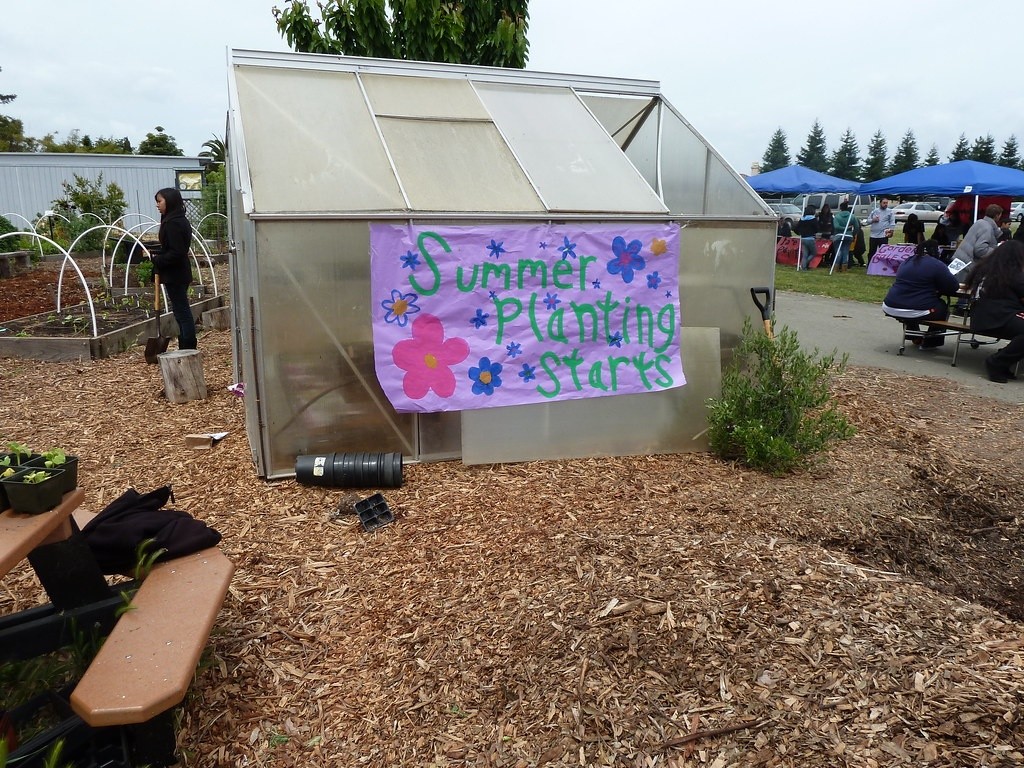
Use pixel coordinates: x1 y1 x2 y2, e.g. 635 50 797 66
152 187 199 351
773 198 1024 384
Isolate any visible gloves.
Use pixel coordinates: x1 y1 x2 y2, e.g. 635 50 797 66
150 255 159 282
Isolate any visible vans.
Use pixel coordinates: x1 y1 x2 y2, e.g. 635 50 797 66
790 191 872 223
924 196 950 210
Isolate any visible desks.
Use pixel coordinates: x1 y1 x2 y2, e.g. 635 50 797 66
946 290 977 369
0 487 113 611
777 236 835 267
867 244 957 277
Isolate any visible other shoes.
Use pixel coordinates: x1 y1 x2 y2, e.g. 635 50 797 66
1005 368 1017 380
912 338 924 344
919 346 939 350
983 355 1008 383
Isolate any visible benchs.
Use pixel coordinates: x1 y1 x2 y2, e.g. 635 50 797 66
885 314 1020 378
0 250 37 280
69 507 234 768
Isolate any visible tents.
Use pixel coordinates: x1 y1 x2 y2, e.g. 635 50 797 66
829 159 1024 274
225 46 780 479
746 164 877 275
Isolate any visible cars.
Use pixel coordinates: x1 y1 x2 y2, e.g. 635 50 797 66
1008 201 1024 222
890 201 945 225
766 203 803 230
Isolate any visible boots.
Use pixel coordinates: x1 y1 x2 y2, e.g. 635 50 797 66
833 262 840 272
842 263 849 273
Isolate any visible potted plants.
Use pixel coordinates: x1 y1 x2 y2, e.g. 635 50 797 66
0 441 79 515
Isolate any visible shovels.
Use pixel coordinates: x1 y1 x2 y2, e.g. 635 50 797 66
144 273 172 364
